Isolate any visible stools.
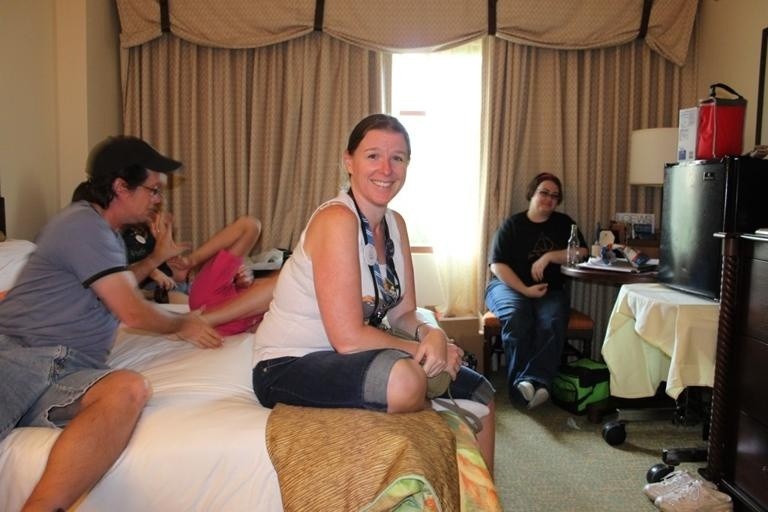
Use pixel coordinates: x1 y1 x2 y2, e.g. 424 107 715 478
478 304 595 386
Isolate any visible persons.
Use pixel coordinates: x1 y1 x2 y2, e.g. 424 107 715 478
484 174 590 411
253 114 498 490
0 76 224 511
166 215 283 336
72 179 194 304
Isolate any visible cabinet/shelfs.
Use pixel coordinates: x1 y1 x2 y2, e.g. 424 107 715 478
697 227 767 510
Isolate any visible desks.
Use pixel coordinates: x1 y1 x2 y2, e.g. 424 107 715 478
560 261 662 289
601 283 719 485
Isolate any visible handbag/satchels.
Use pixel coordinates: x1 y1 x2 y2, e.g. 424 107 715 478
545 355 613 416
374 323 453 402
693 81 748 162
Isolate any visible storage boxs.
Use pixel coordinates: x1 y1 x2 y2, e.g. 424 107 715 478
676 104 699 163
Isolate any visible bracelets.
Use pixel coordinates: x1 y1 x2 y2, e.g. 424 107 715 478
415 322 428 340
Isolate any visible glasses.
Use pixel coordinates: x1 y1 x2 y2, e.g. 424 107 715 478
140 184 163 196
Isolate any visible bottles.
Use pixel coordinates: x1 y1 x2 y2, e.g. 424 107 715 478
567 224 581 267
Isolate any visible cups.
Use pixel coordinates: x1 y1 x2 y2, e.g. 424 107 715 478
591 245 601 256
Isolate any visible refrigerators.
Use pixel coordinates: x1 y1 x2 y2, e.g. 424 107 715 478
657 155 768 302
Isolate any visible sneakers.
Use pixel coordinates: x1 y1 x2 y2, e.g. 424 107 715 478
654 480 735 512
642 470 719 503
527 387 550 410
517 381 535 402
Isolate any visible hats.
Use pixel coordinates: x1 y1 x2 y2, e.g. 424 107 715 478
86 134 182 177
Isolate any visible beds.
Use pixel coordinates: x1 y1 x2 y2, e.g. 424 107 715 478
0 192 501 512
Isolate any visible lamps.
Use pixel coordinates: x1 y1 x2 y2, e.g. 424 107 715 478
406 243 446 318
625 126 679 187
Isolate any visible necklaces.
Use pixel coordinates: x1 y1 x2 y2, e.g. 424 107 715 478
347 186 400 328
